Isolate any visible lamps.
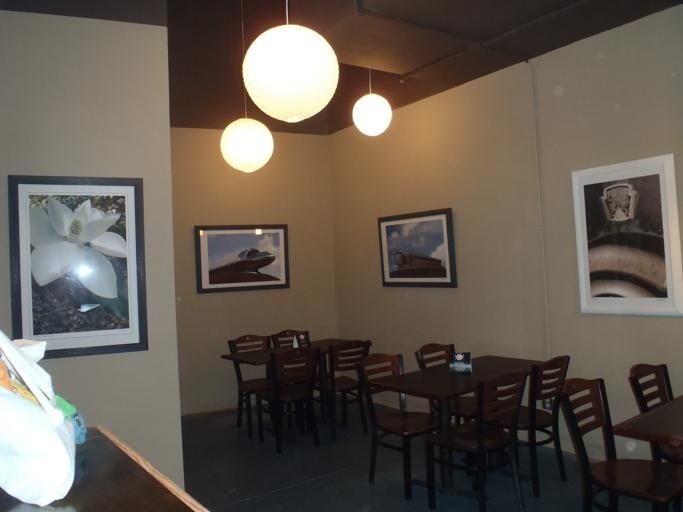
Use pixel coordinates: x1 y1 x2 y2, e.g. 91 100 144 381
220 0 274 173
352 68 392 137
242 0 339 123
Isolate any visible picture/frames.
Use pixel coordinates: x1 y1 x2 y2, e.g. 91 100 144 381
195 225 290 294
572 153 683 318
377 208 457 288
9 175 149 359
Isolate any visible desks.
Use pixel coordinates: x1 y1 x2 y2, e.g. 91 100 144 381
0 425 211 512
